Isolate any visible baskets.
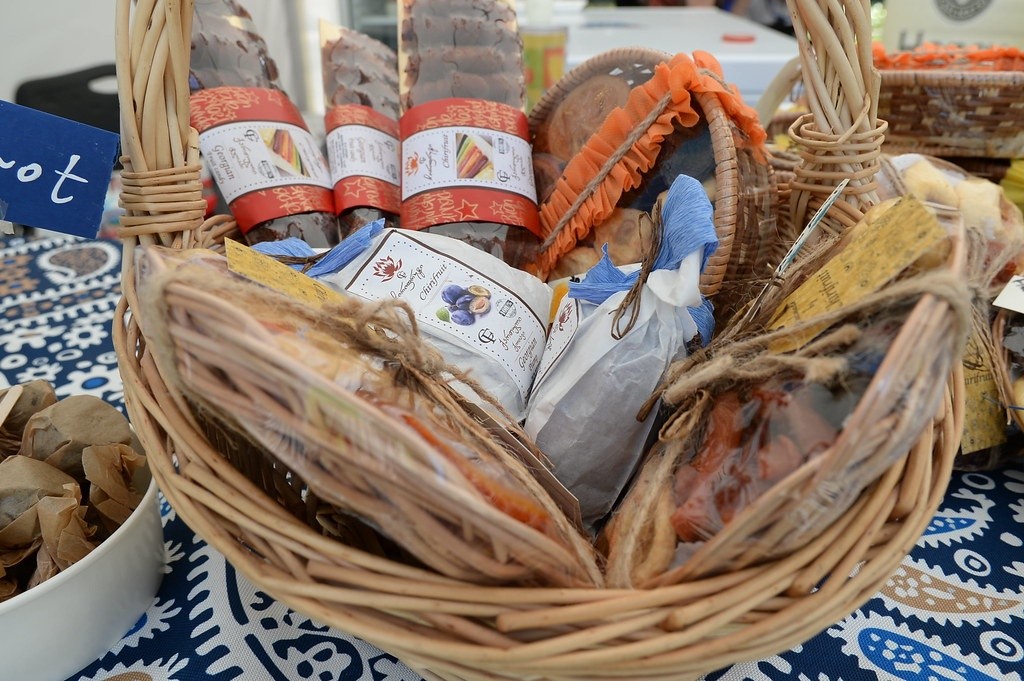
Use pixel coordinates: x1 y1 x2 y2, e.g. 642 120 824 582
979 234 1024 430
878 69 1024 161
113 0 970 681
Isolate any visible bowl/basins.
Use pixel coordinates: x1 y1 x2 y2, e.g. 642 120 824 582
0 423 164 681
